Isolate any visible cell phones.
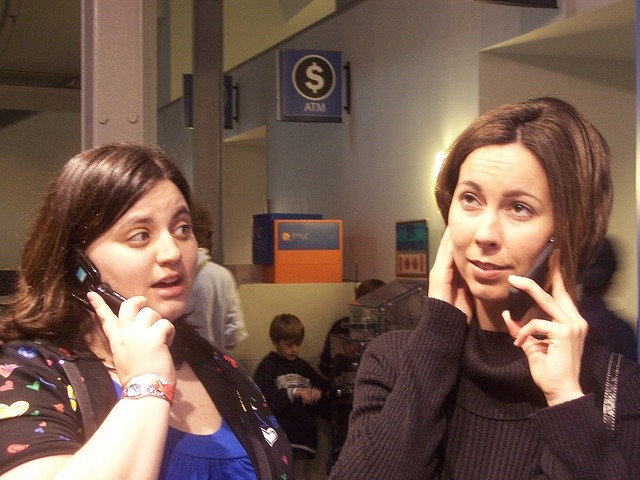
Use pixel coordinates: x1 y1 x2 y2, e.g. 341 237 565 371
509 234 554 319
63 244 127 317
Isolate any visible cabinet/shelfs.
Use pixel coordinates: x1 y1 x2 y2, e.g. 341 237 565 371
339 279 426 371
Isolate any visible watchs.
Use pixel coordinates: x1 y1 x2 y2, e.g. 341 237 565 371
119 376 175 405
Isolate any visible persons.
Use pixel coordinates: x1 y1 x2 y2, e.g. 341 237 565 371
318 279 386 476
181 200 249 352
254 313 327 480
0 142 296 480
329 96 640 479
573 232 638 361
